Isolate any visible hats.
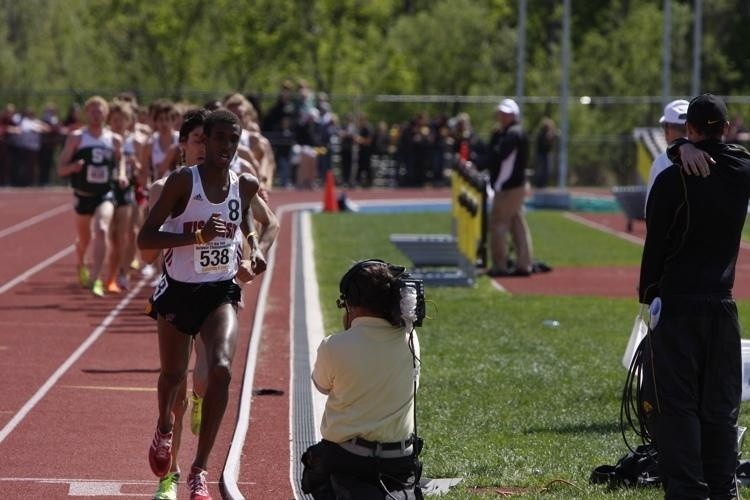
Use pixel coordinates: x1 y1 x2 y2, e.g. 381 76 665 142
496 98 520 115
659 99 689 125
687 93 729 128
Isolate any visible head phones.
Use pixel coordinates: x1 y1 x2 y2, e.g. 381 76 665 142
341 259 385 306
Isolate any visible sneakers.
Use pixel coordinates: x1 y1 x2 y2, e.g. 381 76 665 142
148 416 175 477
152 464 181 500
91 279 105 299
75 264 91 289
186 465 212 500
190 394 203 436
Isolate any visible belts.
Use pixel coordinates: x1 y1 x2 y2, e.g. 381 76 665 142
347 437 413 450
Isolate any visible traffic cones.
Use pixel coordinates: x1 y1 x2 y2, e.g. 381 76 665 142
321 168 339 214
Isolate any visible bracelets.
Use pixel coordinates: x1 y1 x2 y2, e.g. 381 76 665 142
193 225 207 247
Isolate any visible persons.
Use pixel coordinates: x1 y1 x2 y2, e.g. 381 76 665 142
533 117 561 187
637 93 750 498
205 76 483 258
304 258 421 499
58 97 127 297
104 79 184 295
645 98 693 219
0 97 82 188
474 99 535 277
